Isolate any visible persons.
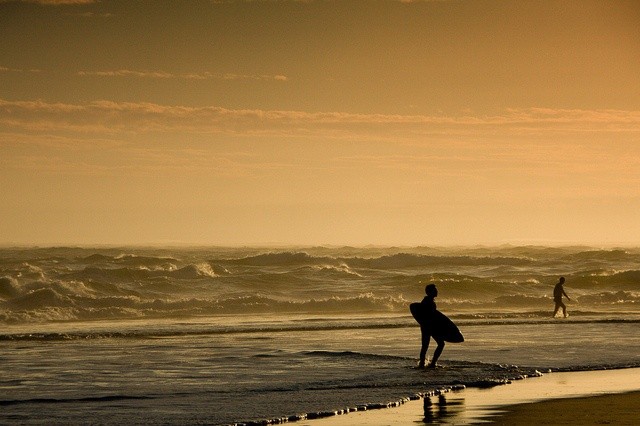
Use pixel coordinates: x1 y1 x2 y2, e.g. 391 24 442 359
552 277 571 318
419 284 445 369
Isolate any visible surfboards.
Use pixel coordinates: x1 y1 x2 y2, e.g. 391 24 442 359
410 303 464 342
